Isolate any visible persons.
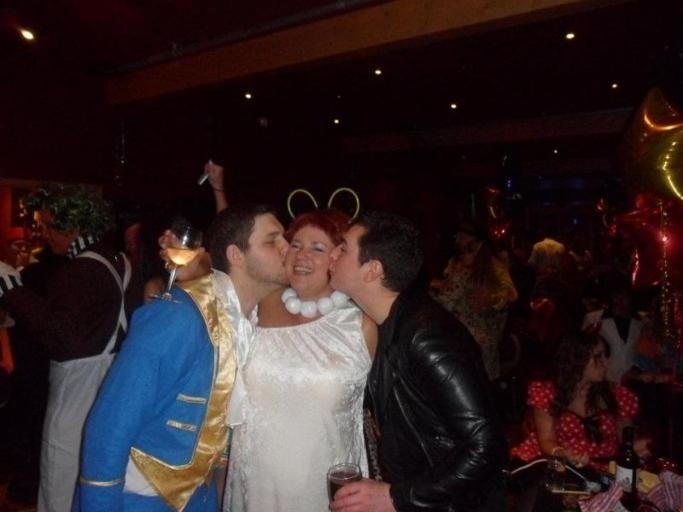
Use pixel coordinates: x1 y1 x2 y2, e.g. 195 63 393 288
0 157 683 512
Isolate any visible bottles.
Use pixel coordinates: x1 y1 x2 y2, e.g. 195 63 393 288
613 425 641 511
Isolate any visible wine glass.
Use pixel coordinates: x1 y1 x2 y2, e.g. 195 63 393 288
150 222 204 304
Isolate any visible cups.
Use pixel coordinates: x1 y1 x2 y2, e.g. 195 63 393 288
328 459 363 506
